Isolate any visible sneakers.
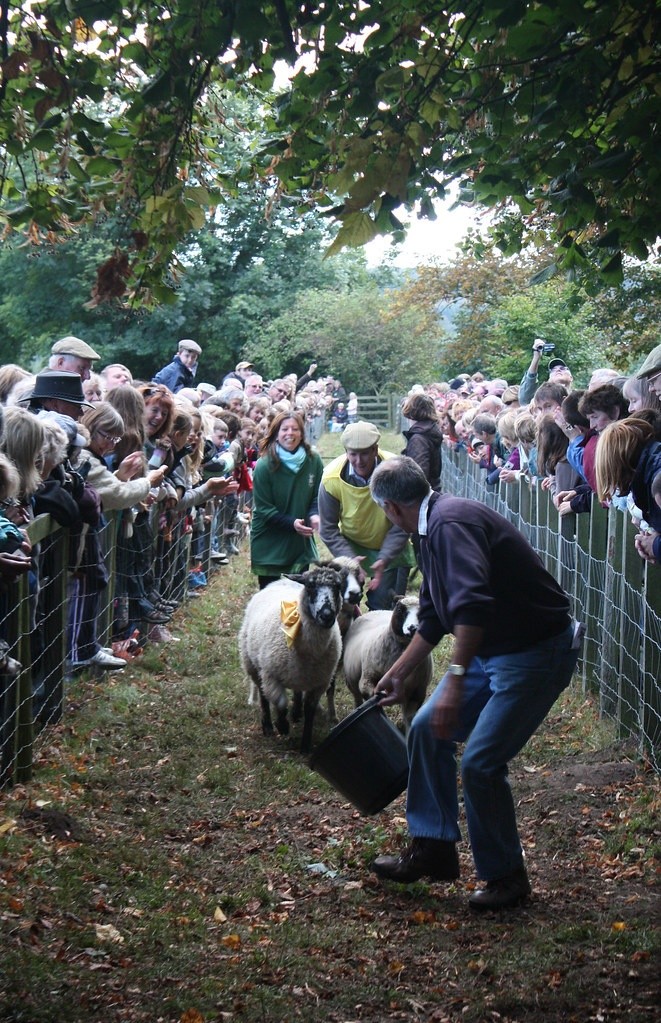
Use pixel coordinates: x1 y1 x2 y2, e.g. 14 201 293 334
373 829 459 881
469 867 531 910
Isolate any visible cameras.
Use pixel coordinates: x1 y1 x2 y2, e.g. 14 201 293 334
537 344 555 352
503 461 514 471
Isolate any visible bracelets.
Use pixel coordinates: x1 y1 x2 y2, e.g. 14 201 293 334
447 662 465 676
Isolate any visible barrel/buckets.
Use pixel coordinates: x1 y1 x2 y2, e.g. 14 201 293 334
307 693 410 816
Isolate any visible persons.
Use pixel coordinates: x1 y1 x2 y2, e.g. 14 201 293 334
252 411 323 594
0 336 661 785
318 421 411 610
153 340 202 396
368 455 578 903
401 394 443 494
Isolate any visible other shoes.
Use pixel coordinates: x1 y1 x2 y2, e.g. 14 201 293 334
66 509 251 670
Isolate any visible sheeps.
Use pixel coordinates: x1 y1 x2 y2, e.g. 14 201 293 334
237 555 366 753
341 590 433 731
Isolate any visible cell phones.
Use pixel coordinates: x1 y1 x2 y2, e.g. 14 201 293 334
149 447 170 471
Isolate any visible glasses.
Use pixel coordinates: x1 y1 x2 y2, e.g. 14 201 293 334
548 366 568 373
506 398 520 406
274 386 288 395
648 372 661 387
95 428 123 444
246 384 262 389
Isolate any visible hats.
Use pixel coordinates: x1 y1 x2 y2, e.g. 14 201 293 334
178 339 202 354
343 420 381 451
197 382 216 396
547 357 568 370
235 361 254 371
44 412 88 448
50 334 101 361
637 344 661 380
16 369 97 410
